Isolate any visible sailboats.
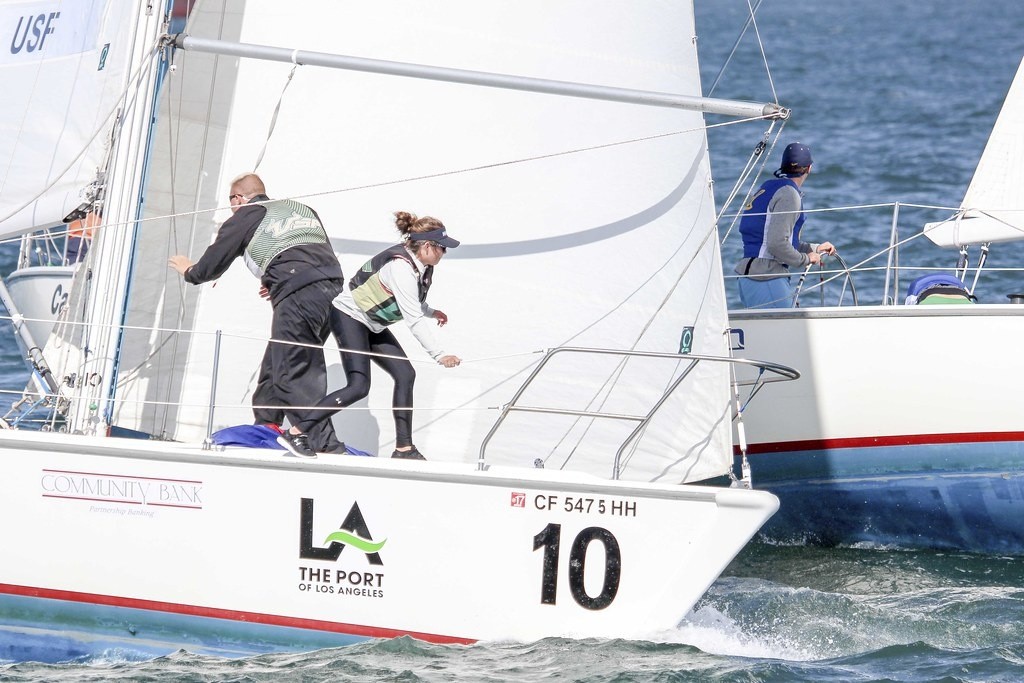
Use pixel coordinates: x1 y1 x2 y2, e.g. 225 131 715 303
713 58 1023 559
1 1 796 651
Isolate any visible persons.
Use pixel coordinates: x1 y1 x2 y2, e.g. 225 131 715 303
734 142 836 309
904 272 976 306
169 173 347 456
277 212 461 461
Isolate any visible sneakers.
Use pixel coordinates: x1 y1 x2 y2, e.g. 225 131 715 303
277 428 318 458
391 444 426 460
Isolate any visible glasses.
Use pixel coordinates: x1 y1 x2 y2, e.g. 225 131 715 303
435 243 446 252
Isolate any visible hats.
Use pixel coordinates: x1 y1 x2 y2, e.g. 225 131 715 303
782 142 813 167
410 226 460 248
